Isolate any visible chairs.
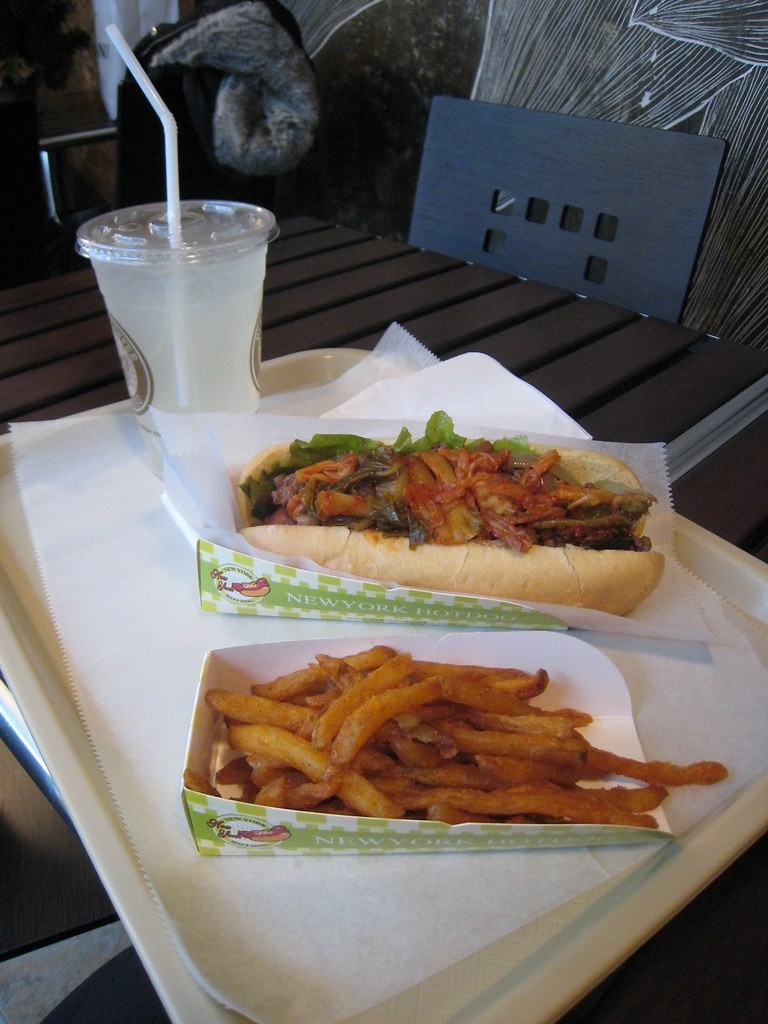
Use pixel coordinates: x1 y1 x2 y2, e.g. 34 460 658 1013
406 96 731 322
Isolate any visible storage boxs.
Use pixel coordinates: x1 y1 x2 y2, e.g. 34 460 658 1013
194 534 573 632
180 632 678 857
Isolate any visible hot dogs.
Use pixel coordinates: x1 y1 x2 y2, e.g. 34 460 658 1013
234 409 665 620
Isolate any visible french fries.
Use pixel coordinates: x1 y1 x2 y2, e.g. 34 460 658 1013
206 645 731 832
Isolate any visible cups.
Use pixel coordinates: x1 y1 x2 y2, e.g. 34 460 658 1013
76 201 277 461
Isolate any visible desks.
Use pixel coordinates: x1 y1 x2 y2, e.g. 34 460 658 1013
0 214 768 485
